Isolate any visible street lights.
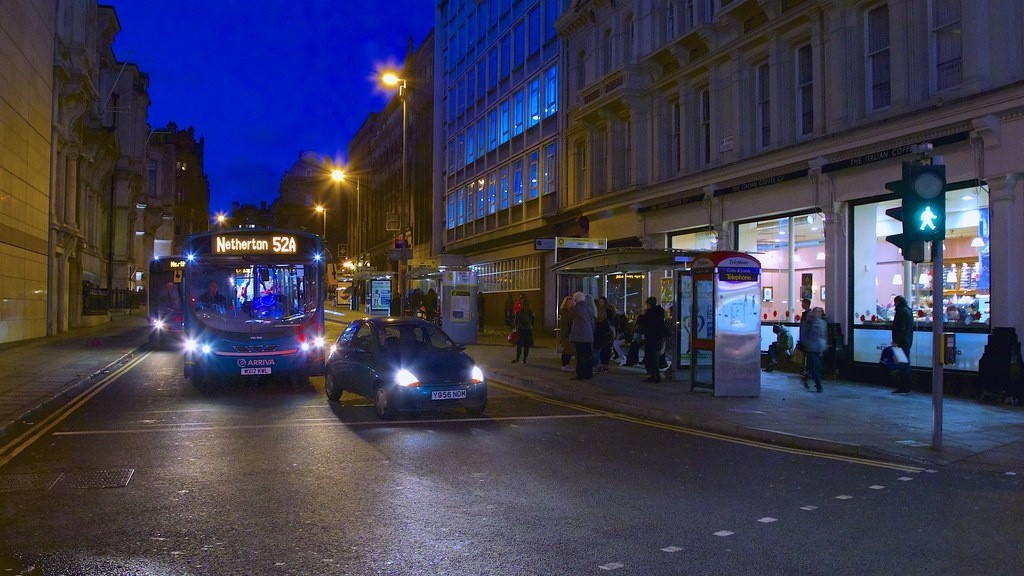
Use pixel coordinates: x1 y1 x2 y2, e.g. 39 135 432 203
333 169 361 273
382 73 407 319
317 205 327 246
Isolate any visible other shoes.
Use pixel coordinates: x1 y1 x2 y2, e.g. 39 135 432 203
800 377 809 389
815 385 823 393
770 361 778 369
512 358 520 363
600 365 609 373
561 366 573 372
891 388 912 395
521 359 527 366
619 361 626 367
622 364 634 368
762 368 773 372
644 377 661 383
573 376 591 382
612 355 620 360
592 368 600 375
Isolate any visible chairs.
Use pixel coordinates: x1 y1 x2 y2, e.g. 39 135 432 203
386 336 400 346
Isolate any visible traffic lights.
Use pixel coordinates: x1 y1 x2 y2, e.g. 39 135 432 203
885 161 926 265
910 164 946 242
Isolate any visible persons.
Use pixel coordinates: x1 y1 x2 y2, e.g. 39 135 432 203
799 299 827 393
477 292 485 332
762 324 793 371
504 292 522 328
891 296 913 396
510 300 535 363
378 327 387 346
559 292 668 383
200 280 226 307
411 288 437 320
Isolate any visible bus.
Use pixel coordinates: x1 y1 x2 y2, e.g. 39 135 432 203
173 227 339 393
141 256 187 343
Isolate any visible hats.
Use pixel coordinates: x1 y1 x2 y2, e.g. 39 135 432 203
773 324 782 333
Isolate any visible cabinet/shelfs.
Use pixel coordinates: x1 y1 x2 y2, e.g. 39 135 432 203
915 258 980 294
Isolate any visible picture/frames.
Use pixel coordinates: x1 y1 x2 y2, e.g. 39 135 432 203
802 273 814 287
820 286 826 301
800 287 813 300
763 286 773 301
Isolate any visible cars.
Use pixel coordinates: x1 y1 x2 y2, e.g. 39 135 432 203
324 316 488 421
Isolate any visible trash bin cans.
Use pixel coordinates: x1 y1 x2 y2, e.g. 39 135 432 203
349 296 358 311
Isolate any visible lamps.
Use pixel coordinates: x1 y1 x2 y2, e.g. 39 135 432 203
756 214 814 231
970 188 984 247
794 235 801 264
892 269 903 285
817 223 826 260
947 272 958 283
919 273 929 285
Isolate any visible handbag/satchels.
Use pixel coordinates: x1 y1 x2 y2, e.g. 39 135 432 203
892 345 909 365
506 329 521 346
789 344 807 367
878 346 898 370
